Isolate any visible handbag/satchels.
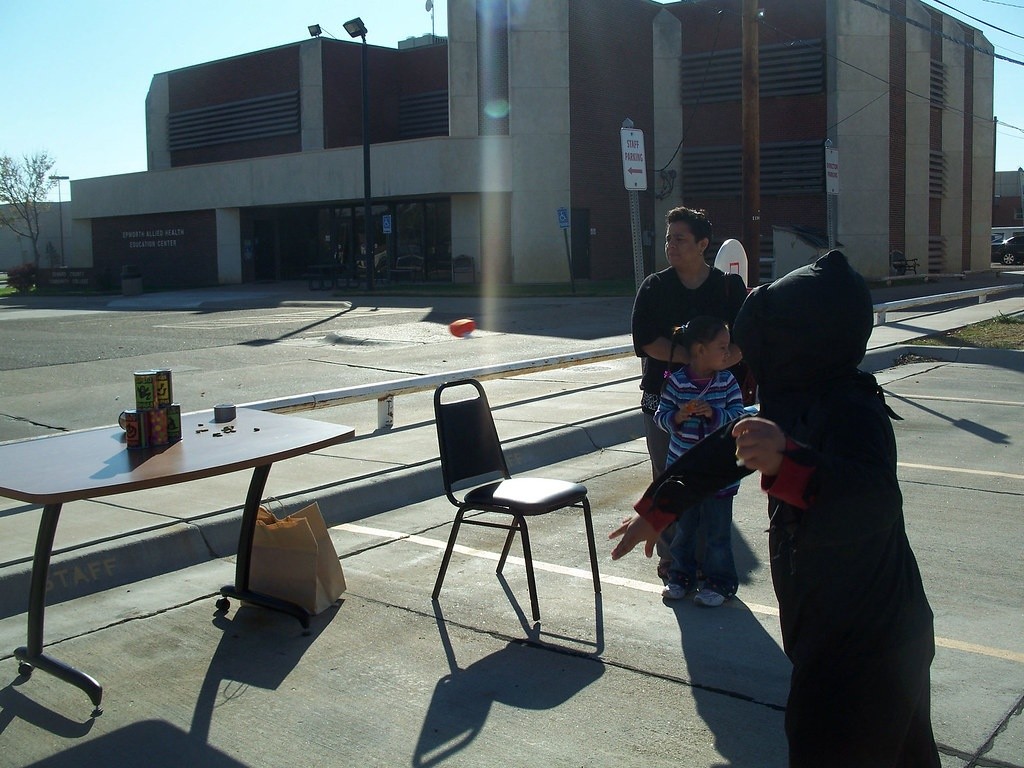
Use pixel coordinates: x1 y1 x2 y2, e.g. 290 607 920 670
248 498 346 615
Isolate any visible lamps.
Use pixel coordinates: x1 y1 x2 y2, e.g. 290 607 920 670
307 24 323 38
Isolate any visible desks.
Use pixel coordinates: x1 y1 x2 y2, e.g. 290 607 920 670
306 264 345 288
0 405 356 717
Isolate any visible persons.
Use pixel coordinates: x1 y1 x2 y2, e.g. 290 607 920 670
652 315 744 607
632 207 747 581
609 251 942 768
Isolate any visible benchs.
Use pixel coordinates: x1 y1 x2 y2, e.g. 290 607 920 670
890 250 920 275
388 254 426 284
301 273 360 281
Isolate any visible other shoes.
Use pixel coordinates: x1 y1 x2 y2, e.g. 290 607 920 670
657 560 670 579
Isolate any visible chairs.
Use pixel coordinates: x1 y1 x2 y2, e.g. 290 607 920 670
431 379 601 622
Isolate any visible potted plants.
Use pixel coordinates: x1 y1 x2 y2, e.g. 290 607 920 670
119 264 143 297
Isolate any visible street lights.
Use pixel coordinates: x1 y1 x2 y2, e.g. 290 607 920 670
49 175 69 266
343 17 376 292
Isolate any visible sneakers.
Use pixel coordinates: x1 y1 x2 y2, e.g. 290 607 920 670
695 587 736 606
662 576 706 599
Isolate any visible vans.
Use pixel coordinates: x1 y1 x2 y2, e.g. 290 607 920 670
991 226 1024 245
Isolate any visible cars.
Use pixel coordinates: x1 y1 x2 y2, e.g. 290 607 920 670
992 234 1024 265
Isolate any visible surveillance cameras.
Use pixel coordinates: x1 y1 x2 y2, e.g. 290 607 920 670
825 140 832 148
